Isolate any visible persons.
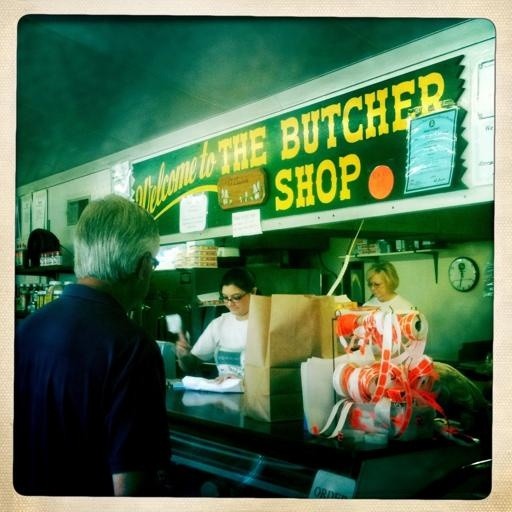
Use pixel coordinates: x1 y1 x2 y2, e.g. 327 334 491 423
177 270 259 384
16 194 173 496
353 262 414 312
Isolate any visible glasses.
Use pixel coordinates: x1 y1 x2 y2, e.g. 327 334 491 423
219 292 247 301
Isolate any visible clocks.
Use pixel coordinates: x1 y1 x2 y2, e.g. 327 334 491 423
449 257 480 292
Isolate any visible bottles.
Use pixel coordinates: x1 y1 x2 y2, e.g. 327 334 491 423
39 251 62 268
15 281 72 314
